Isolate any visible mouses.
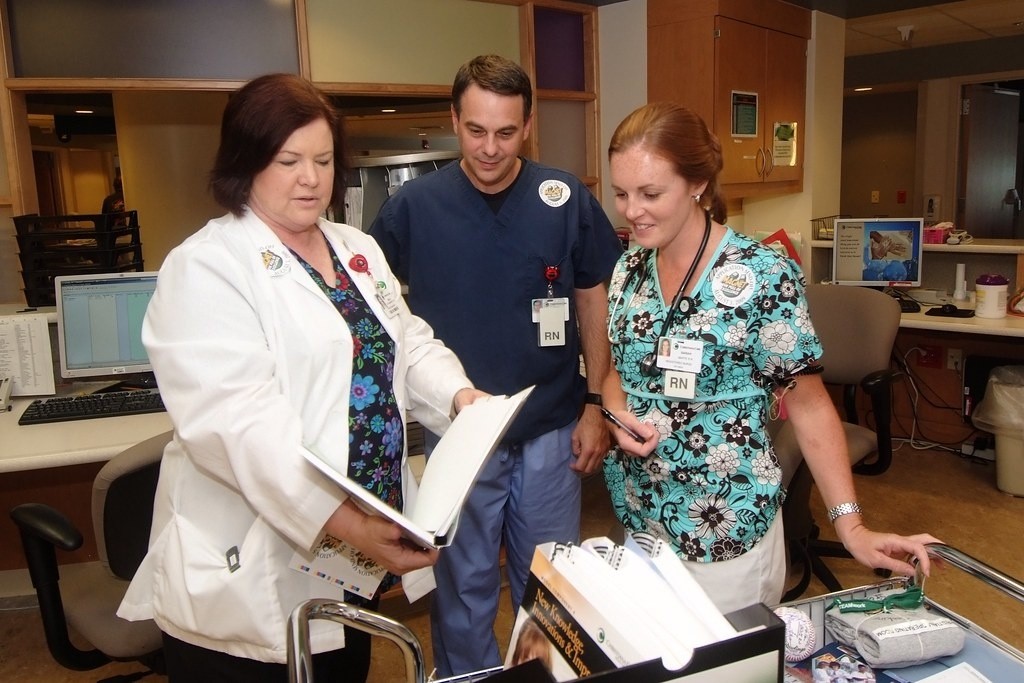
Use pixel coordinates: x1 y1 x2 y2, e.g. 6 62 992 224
941 304 957 314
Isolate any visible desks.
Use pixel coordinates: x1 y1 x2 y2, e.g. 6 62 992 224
0 380 176 472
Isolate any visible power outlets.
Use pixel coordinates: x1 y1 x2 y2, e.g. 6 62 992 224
947 347 962 370
916 343 944 369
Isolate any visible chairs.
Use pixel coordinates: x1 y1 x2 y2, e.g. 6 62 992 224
776 283 902 603
10 428 174 682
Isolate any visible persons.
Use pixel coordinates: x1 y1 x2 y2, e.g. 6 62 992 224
375 295 388 308
102 175 126 228
870 231 906 260
813 662 873 683
533 300 543 313
364 55 610 683
116 74 493 683
659 338 670 357
601 100 947 614
510 617 552 673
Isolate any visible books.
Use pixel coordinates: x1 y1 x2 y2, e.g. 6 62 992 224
298 385 536 551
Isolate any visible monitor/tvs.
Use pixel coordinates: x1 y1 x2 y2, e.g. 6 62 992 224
54 271 161 388
832 217 924 291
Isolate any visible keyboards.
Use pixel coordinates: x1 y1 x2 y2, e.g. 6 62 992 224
896 300 921 312
18 386 167 425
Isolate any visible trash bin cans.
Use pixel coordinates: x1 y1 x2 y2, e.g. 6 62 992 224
990 366 1024 498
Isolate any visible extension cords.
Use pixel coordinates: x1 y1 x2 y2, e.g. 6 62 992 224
961 441 996 461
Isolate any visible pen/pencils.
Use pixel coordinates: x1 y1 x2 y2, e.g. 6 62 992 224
599 407 665 461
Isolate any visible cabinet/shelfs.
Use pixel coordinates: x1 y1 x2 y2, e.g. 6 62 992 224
646 0 813 200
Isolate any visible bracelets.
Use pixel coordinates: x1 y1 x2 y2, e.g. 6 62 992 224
872 255 882 260
829 503 865 523
585 393 603 407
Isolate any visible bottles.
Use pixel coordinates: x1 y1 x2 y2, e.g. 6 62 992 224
975 274 1010 318
